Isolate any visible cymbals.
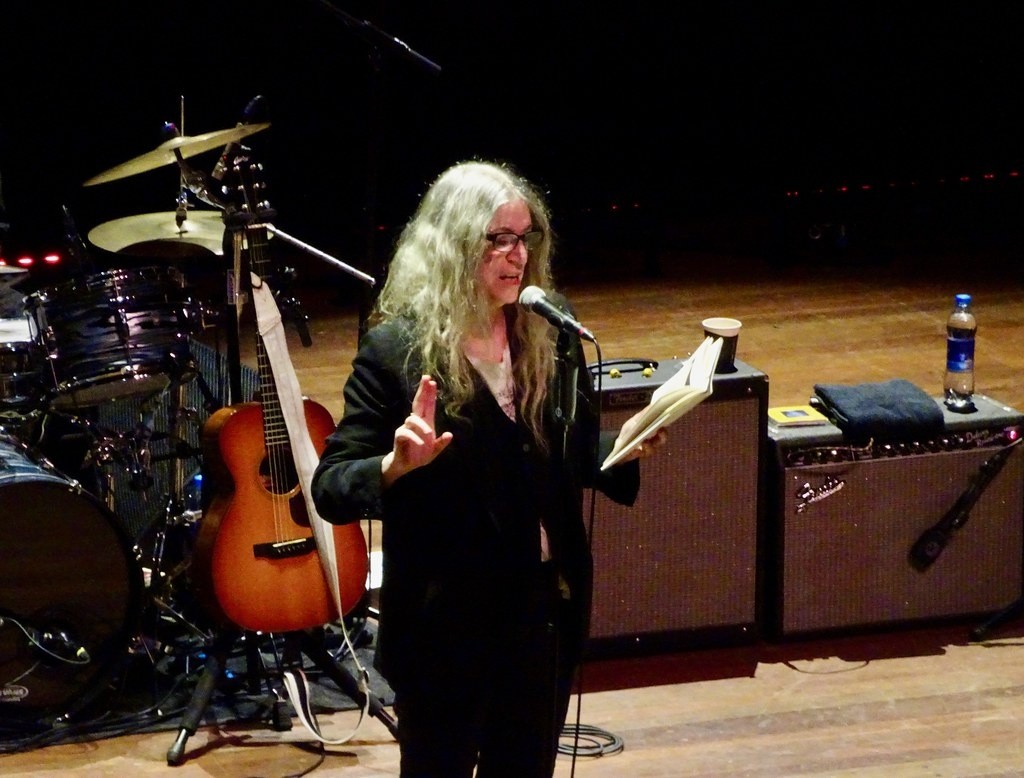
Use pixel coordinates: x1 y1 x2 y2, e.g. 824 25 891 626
84 122 274 187
88 209 275 255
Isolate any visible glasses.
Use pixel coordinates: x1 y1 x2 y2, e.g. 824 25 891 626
486 232 544 252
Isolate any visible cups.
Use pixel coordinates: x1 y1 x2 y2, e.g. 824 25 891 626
700 317 743 371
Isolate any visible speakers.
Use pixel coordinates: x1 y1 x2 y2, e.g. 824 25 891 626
581 357 1024 662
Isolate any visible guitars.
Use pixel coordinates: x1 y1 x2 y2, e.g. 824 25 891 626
191 142 373 635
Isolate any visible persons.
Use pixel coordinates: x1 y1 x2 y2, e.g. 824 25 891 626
310 160 669 777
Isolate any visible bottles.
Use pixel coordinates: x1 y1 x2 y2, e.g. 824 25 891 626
942 293 979 410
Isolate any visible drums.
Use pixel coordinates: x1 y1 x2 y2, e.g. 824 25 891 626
0 425 140 738
15 265 200 412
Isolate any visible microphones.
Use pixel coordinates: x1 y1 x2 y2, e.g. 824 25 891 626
516 285 594 341
